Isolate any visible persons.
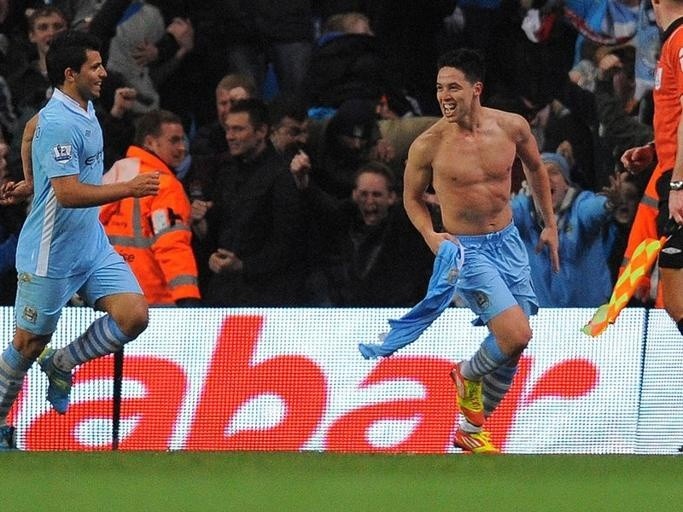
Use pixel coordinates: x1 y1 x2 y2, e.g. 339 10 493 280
403 48 559 455
0 31 160 451
1 1 683 335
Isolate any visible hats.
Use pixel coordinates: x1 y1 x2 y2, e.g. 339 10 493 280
334 99 375 140
540 152 571 184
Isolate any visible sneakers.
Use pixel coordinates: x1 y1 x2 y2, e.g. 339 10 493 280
454 427 500 455
449 359 485 426
37 348 71 414
0 424 14 451
